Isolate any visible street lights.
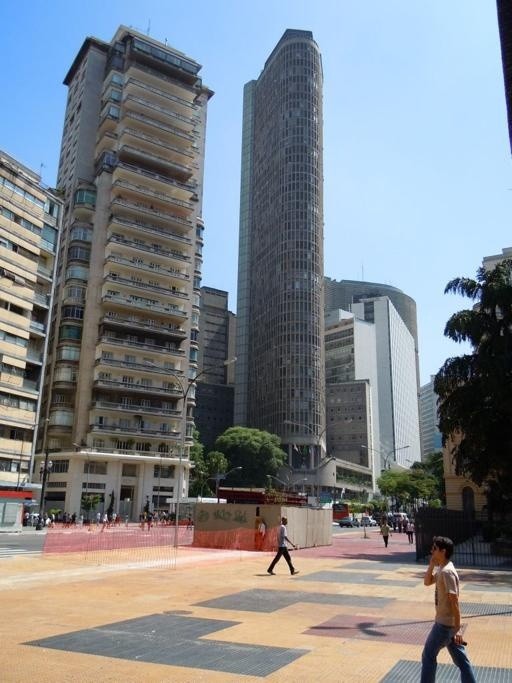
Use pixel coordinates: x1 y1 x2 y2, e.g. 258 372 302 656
284 418 353 507
213 467 244 497
16 417 50 492
266 473 307 492
144 357 238 549
73 442 91 502
35 460 54 531
360 445 410 503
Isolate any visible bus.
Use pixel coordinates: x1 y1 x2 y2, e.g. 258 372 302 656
334 502 369 523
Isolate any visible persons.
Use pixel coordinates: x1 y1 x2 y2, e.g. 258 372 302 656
380 516 415 548
257 519 266 551
266 516 300 576
23 510 169 533
419 534 478 683
185 513 192 530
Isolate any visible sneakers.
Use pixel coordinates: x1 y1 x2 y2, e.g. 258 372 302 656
293 571 299 575
267 569 275 575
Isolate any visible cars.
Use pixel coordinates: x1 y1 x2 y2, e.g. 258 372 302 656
339 512 409 528
